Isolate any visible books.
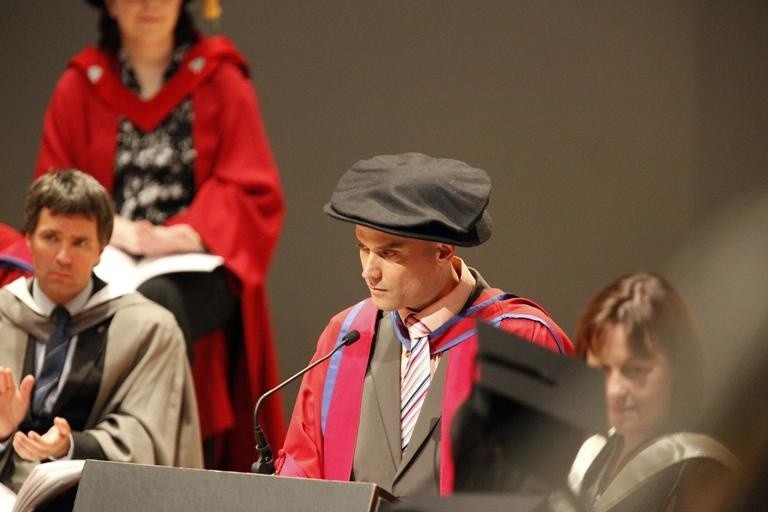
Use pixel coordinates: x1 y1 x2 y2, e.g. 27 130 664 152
0 457 83 511
86 243 225 297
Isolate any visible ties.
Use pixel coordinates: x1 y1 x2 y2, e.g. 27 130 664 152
32 308 70 416
398 316 430 454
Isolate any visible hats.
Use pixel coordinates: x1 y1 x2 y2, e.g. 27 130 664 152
323 151 494 247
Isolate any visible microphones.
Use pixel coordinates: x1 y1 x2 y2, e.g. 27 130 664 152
251 328 361 474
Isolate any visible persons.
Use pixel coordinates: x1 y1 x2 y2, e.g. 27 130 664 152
270 150 577 496
34 0 286 477
0 168 206 511
557 271 754 512
